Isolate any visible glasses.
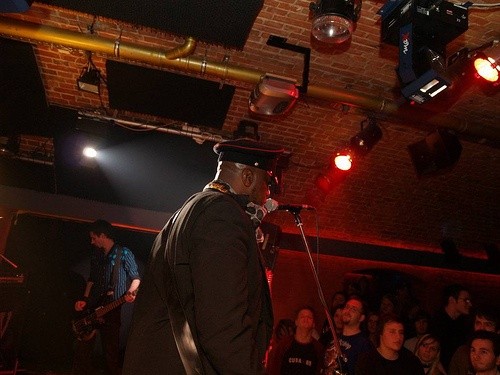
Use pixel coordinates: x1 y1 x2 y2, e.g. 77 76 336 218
458 297 471 303
345 304 361 313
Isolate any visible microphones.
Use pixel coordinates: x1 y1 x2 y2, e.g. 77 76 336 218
265 197 315 212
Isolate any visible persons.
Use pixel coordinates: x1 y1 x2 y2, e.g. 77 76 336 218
122 136 291 375
263 276 500 375
72 219 140 375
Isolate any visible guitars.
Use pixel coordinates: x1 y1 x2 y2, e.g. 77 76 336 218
71 288 139 341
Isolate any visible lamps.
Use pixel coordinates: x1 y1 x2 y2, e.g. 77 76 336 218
306 0 363 46
409 131 463 177
75 65 103 96
474 42 500 85
334 121 385 172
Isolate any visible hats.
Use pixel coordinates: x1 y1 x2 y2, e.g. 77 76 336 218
216 137 285 173
